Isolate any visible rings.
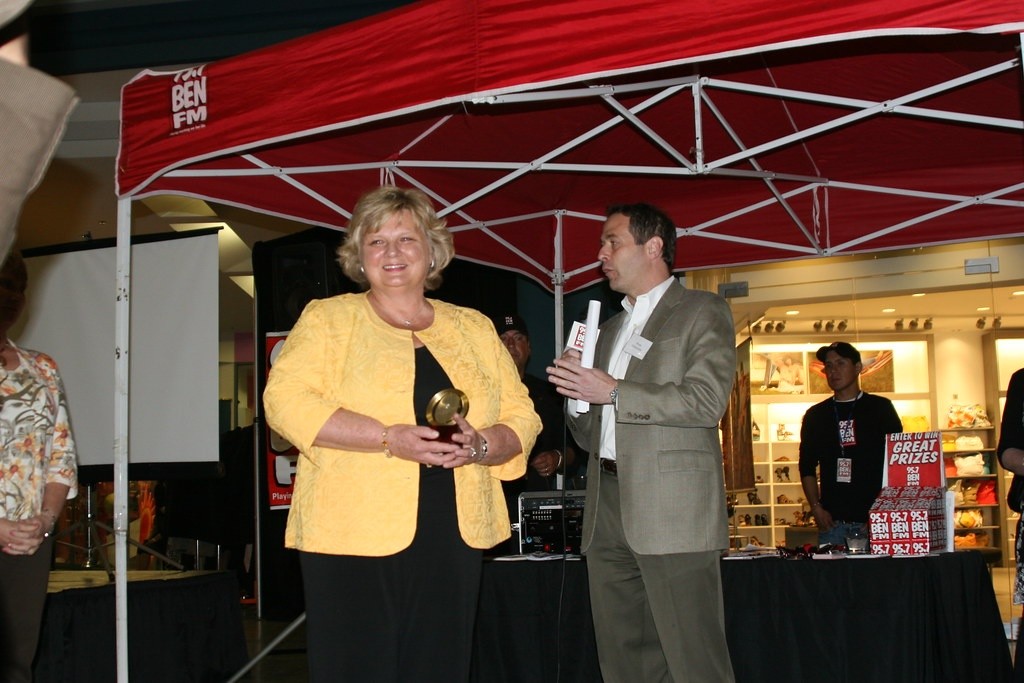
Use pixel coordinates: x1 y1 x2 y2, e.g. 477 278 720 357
547 472 550 476
471 447 477 457
545 467 548 473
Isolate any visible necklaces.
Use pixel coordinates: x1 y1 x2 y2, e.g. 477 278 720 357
370 292 426 326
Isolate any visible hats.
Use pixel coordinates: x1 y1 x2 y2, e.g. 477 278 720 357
816 342 861 363
496 314 530 337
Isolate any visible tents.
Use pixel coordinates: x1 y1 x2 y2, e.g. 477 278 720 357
114 1 1024 683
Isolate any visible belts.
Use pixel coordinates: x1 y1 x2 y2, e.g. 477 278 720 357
600 458 618 476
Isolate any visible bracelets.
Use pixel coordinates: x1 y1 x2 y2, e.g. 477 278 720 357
43 509 55 537
478 438 487 462
382 424 392 457
610 388 618 403
810 502 820 508
554 449 563 469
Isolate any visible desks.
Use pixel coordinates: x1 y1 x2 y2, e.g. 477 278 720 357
472 551 1015 683
27 567 250 683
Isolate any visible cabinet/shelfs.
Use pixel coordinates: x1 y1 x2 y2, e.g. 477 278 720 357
729 399 1019 571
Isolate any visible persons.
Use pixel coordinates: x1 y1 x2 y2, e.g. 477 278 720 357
778 356 803 388
799 342 903 542
0 255 78 683
996 368 1024 604
545 201 735 683
492 312 578 547
264 186 543 683
